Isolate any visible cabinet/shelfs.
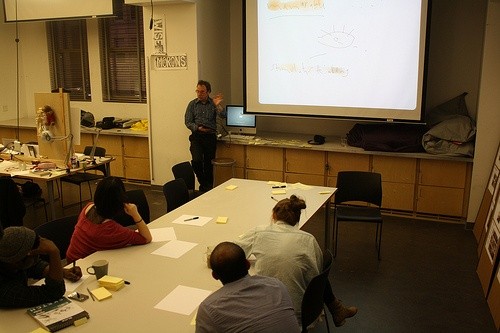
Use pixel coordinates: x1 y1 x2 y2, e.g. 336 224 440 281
327 151 370 207
373 155 416 212
212 142 245 180
0 127 151 185
419 159 468 217
284 149 326 187
246 145 284 182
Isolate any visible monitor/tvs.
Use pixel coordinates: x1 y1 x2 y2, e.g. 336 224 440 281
225 105 256 134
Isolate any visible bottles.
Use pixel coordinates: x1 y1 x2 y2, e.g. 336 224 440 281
72 149 77 170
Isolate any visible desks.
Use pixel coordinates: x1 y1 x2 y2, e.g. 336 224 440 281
0 149 116 222
0 176 338 333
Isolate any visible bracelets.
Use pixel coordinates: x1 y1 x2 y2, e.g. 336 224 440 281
135 218 143 224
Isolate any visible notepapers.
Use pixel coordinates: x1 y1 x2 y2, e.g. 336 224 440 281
272 190 286 195
217 216 228 224
226 185 238 191
98 275 125 291
91 287 112 302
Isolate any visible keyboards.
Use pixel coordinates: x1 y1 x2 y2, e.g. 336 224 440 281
225 134 255 140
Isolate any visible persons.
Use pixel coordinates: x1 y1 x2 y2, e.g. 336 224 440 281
234 194 358 327
0 177 82 310
196 242 301 333
184 79 226 192
66 176 152 266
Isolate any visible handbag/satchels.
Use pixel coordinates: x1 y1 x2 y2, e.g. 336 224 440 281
80 110 94 127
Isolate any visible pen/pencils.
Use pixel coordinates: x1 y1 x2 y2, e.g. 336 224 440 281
72 259 76 273
86 287 95 301
272 185 286 188
184 217 199 221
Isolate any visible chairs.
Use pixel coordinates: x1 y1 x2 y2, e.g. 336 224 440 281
0 147 202 281
300 248 335 333
334 170 384 263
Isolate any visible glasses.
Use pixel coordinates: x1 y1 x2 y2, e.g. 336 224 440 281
195 89 208 94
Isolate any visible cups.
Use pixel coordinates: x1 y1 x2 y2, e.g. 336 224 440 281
87 260 109 279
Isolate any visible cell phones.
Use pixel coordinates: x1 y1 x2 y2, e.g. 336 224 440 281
68 292 89 302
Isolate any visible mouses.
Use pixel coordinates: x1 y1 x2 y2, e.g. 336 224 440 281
255 138 261 142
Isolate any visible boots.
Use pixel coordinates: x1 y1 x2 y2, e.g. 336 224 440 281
327 297 357 327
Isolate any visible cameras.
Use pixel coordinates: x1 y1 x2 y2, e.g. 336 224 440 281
314 135 325 144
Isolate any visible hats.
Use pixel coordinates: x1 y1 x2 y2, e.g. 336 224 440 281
0 227 36 263
